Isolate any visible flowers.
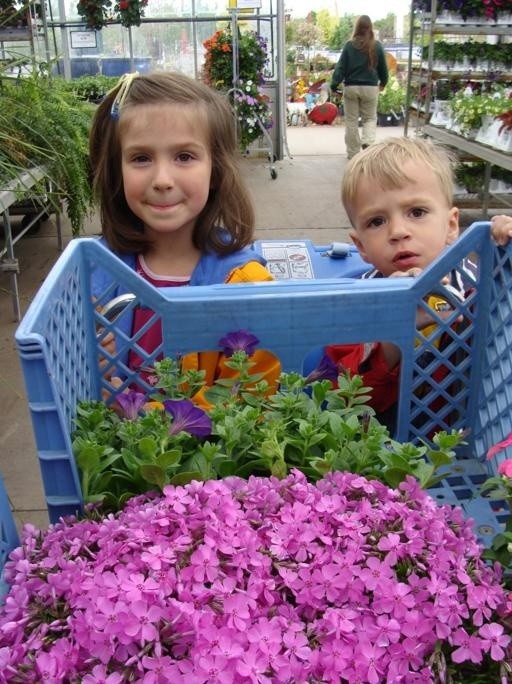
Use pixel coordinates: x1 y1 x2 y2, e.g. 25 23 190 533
200 29 274 160
0 466 512 683
114 0 148 28
114 388 145 427
158 398 213 461
77 0 112 30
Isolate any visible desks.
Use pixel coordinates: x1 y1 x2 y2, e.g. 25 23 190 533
50 56 152 79
0 148 83 321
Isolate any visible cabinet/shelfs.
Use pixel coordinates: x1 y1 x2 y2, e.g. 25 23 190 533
0 0 51 96
402 0 512 294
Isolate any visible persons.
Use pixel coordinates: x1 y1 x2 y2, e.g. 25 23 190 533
317 134 512 461
84 70 281 419
329 13 389 160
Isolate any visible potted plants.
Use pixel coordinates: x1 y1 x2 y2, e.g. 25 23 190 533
378 80 405 126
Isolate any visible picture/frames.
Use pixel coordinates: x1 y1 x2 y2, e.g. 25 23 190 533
70 30 97 48
